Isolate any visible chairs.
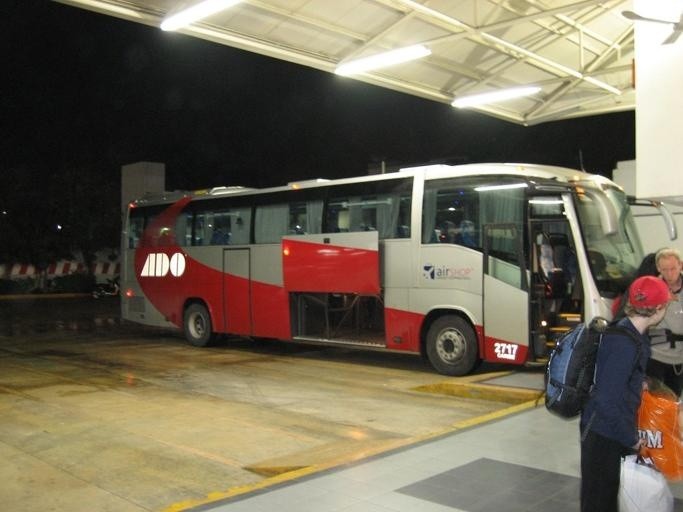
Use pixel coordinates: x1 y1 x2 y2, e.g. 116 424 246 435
396 224 410 237
432 220 486 250
549 233 580 286
187 227 234 245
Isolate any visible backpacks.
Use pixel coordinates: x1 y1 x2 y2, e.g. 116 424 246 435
544 318 641 419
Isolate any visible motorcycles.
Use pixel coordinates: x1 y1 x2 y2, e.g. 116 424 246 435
93 275 119 299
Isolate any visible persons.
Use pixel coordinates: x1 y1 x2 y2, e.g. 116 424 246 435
579 276 678 512
646 248 682 394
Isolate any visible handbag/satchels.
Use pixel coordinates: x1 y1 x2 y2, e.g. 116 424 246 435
637 376 682 482
616 443 673 512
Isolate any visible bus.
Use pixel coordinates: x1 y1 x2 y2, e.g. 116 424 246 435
117 160 679 378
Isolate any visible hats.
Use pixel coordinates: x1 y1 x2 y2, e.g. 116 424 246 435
628 276 673 306
